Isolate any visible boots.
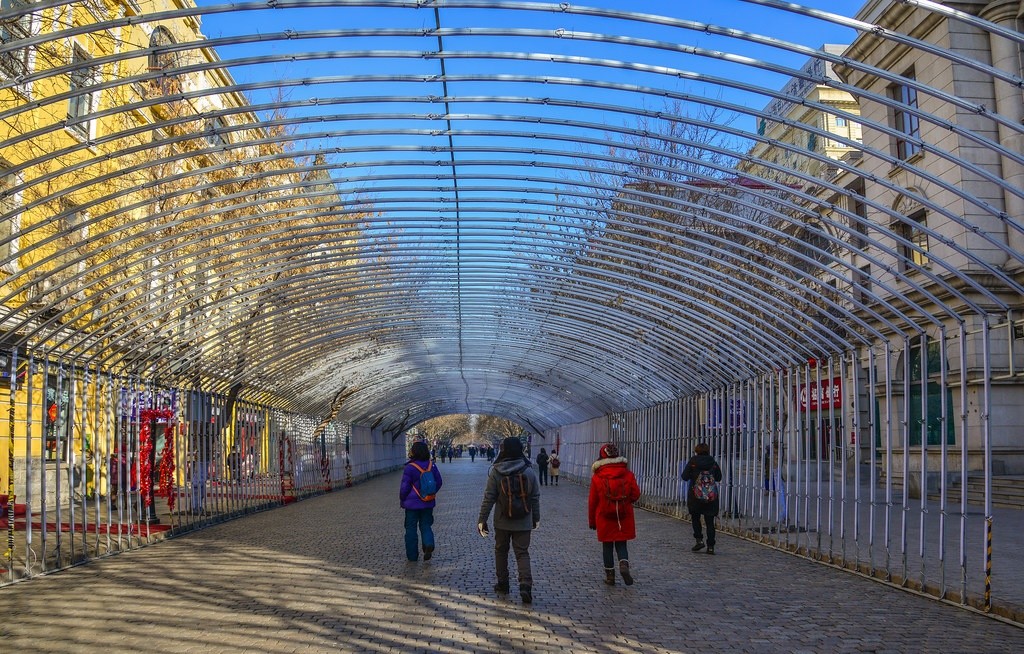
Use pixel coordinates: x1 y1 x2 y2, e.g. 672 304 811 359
619 558 633 586
602 568 615 586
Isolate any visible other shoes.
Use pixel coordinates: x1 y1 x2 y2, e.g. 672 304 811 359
494 584 508 594
520 584 531 604
555 482 558 486
692 538 704 551
707 544 714 554
551 482 553 485
423 544 433 560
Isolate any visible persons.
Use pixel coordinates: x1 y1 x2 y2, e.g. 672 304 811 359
448 448 454 463
242 446 253 483
487 447 495 463
470 447 476 462
546 449 561 486
440 445 446 463
681 443 723 554
227 446 244 487
399 442 443 560
477 436 541 603
588 444 640 586
764 445 775 497
536 448 549 485
432 447 436 462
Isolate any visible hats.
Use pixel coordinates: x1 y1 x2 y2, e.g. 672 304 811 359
492 437 531 466
599 444 618 459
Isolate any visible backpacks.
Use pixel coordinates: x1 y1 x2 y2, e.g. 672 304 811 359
409 460 436 502
602 476 625 520
693 462 717 504
550 455 560 468
497 464 531 520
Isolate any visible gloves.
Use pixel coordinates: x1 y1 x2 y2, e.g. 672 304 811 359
532 520 540 530
477 522 489 538
